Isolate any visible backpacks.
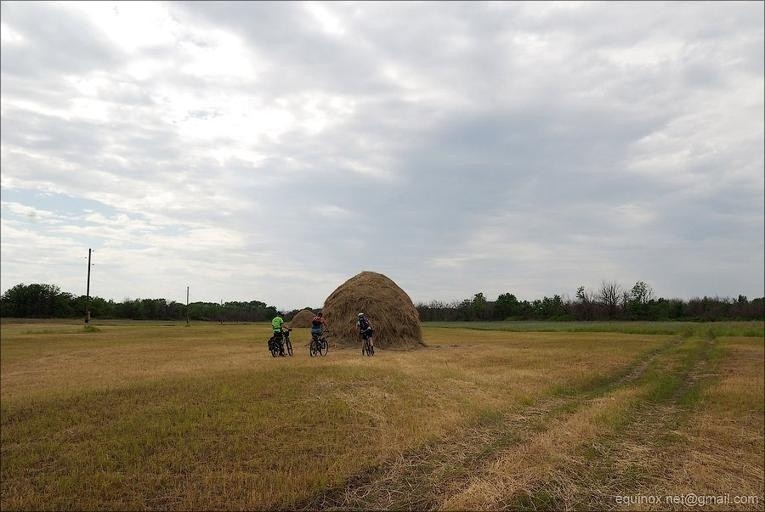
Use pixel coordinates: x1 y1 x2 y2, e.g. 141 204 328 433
312 316 320 325
268 337 279 350
359 318 369 330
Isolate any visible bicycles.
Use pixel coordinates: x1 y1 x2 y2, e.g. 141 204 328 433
309 329 330 357
356 327 375 357
267 327 294 358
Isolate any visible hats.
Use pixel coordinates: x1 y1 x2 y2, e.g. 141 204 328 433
358 313 364 316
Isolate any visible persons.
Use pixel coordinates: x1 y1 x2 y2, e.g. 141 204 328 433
311 313 328 354
272 310 291 356
356 313 374 355
